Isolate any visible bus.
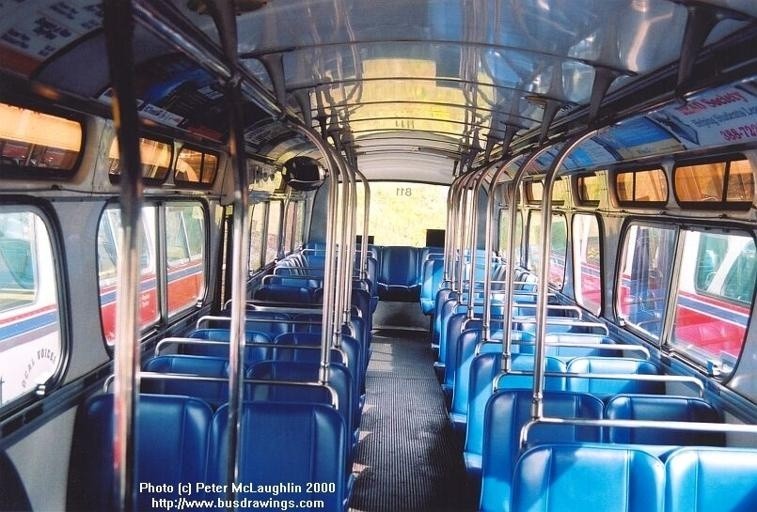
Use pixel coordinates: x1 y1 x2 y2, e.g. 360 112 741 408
0 207 205 405
536 211 755 378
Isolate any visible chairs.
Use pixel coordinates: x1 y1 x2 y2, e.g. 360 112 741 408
66 243 378 509
421 247 755 511
377 247 419 300
